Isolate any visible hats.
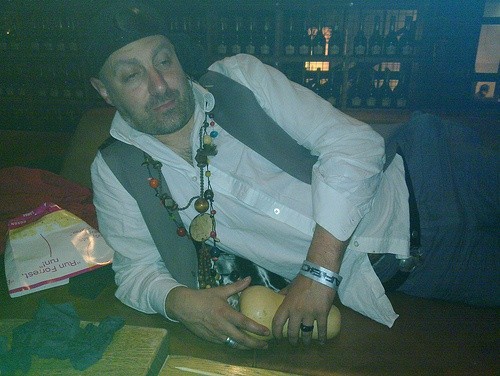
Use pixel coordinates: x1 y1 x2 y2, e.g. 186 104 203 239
82 0 168 77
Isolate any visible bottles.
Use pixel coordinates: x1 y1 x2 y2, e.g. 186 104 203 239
0 8 81 54
214 12 272 55
348 69 361 107
365 68 377 108
165 6 204 33
399 15 415 56
312 15 326 55
328 15 342 55
285 67 338 107
353 15 367 56
385 15 398 56
394 67 409 110
379 67 393 109
283 15 311 56
369 15 383 55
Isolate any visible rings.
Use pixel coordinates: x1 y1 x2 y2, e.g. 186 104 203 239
299 323 315 333
224 336 236 350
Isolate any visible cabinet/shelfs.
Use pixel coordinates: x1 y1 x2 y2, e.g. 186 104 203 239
0 0 424 124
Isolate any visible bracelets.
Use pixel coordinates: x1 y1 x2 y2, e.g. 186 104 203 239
299 260 344 291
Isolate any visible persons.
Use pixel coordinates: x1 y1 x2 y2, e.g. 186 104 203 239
81 1 500 352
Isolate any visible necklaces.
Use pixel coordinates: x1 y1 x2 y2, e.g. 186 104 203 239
140 109 225 289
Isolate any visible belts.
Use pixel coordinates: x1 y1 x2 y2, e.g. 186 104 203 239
382 146 422 294
